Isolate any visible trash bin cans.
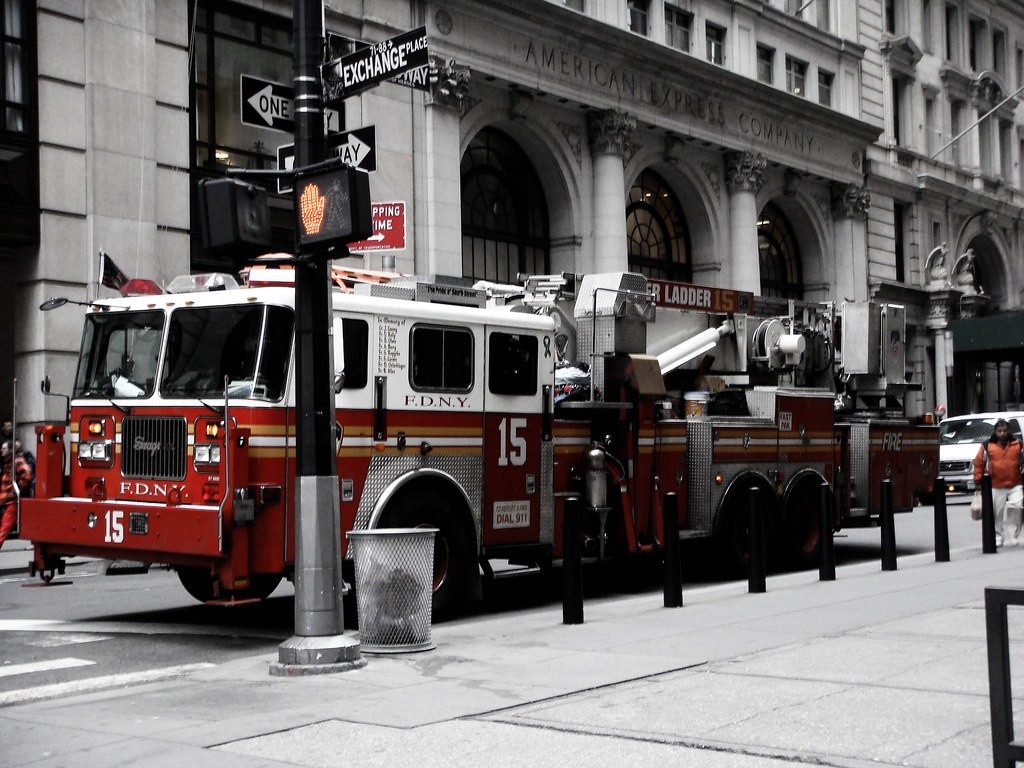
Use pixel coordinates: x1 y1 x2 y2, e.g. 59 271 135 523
348 525 439 653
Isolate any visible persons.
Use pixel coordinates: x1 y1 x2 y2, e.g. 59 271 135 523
0 420 36 551
973 420 1024 548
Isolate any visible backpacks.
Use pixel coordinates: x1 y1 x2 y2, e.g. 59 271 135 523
14 451 37 488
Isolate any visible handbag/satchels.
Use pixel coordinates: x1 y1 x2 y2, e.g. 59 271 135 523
969 490 982 521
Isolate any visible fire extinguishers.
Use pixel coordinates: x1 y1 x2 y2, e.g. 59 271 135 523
582 435 612 508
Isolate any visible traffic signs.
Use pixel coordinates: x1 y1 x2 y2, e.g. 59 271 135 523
239 72 349 136
277 122 379 194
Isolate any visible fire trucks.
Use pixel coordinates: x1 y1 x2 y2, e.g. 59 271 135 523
17 246 947 627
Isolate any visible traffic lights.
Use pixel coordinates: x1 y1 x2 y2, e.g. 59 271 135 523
293 155 374 249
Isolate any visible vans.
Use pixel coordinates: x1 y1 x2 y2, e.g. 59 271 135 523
930 411 1024 494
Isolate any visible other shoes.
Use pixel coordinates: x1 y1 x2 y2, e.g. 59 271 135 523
1010 537 1020 547
996 534 1004 548
